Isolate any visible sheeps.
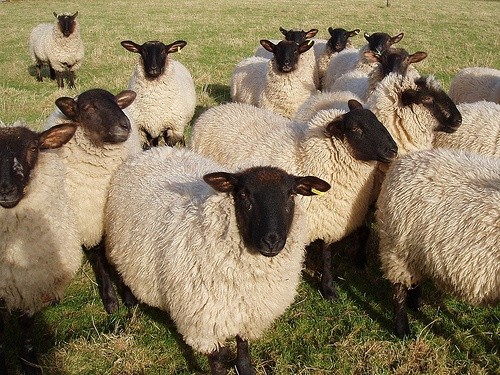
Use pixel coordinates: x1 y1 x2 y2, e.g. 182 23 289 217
228 39 317 118
103 147 332 375
120 40 195 149
28 11 86 90
39 88 141 314
192 97 399 302
372 149 500 338
0 122 83 375
257 24 500 209
